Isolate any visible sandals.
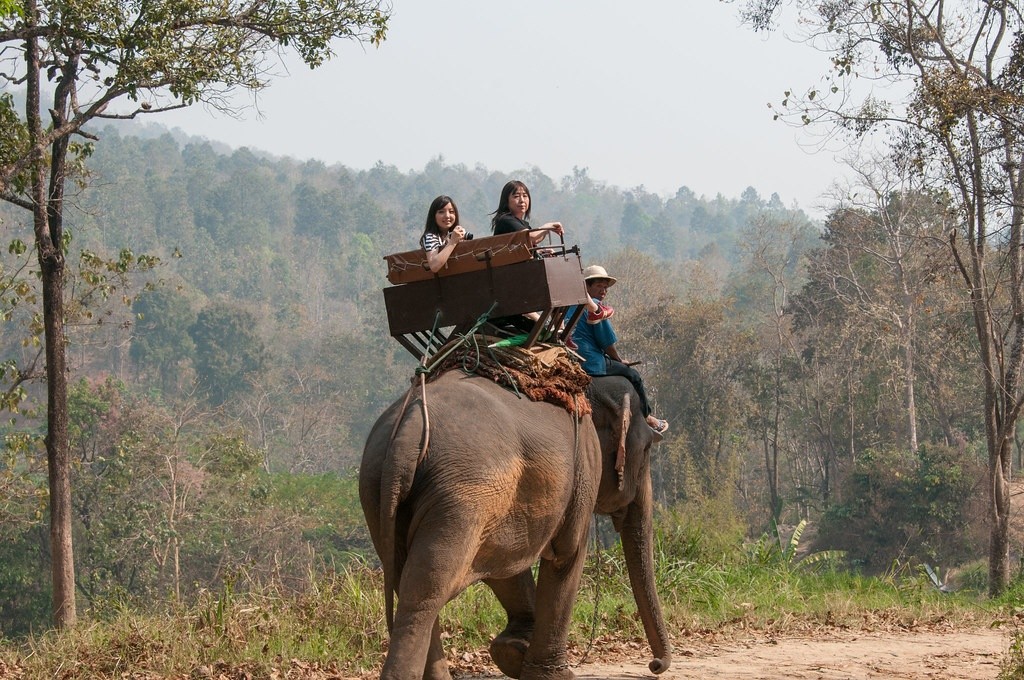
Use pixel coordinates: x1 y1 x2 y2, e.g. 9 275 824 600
648 419 668 443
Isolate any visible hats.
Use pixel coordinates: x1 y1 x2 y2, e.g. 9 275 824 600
584 265 617 287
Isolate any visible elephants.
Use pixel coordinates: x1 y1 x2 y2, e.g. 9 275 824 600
357 369 674 680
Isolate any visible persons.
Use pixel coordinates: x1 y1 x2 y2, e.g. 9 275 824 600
565 265 669 433
419 195 466 273
487 181 615 351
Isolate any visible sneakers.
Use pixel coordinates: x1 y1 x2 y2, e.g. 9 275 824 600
566 333 578 350
587 301 614 324
